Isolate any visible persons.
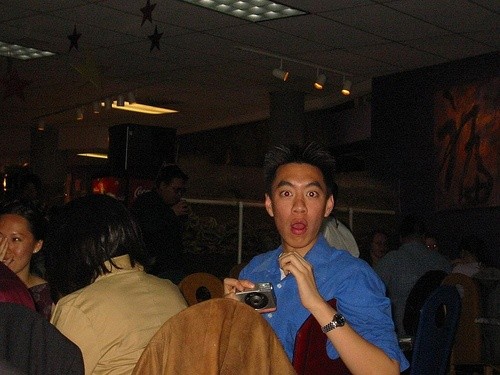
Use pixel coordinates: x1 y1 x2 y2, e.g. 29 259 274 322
12 175 51 237
223 140 410 375
132 162 193 282
360 214 500 341
50 195 188 375
0 205 58 320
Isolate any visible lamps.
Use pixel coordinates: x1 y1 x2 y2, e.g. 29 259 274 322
31 90 179 132
235 47 353 96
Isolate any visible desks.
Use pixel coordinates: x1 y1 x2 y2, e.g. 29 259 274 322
178 196 395 265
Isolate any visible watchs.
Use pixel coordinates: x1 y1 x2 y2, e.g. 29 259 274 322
320 313 345 333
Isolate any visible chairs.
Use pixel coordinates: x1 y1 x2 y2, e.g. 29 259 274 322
131 270 500 375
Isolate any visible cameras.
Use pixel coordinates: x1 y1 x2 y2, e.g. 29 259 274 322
235 282 276 310
